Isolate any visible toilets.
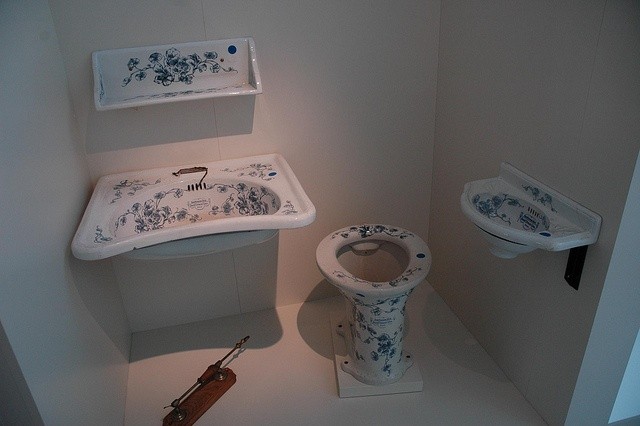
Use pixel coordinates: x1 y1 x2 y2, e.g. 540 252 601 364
316 224 431 398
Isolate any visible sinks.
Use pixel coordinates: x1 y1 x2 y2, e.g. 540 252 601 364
462 162 602 251
70 153 317 262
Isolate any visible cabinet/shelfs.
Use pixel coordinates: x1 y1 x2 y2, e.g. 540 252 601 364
91 36 262 111
